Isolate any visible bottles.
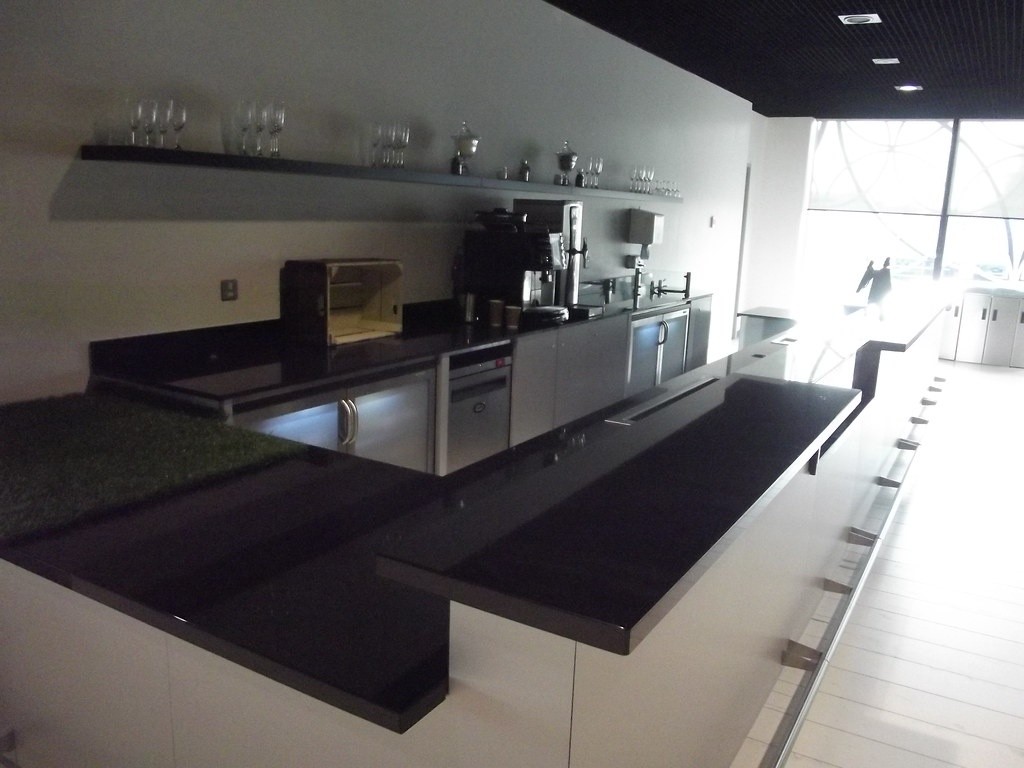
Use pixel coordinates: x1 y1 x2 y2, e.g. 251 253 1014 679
503 166 508 180
575 168 585 187
554 173 567 185
521 160 530 182
451 149 463 175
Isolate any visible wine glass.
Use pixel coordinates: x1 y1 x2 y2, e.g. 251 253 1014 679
629 164 680 198
584 157 603 188
239 98 286 160
123 98 188 152
369 119 411 171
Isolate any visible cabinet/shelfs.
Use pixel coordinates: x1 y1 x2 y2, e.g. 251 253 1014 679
937 288 1024 367
232 369 434 476
628 308 689 399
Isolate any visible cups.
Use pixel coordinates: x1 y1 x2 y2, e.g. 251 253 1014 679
505 305 522 329
489 300 503 326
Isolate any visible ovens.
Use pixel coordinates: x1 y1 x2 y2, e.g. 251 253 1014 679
435 339 510 485
